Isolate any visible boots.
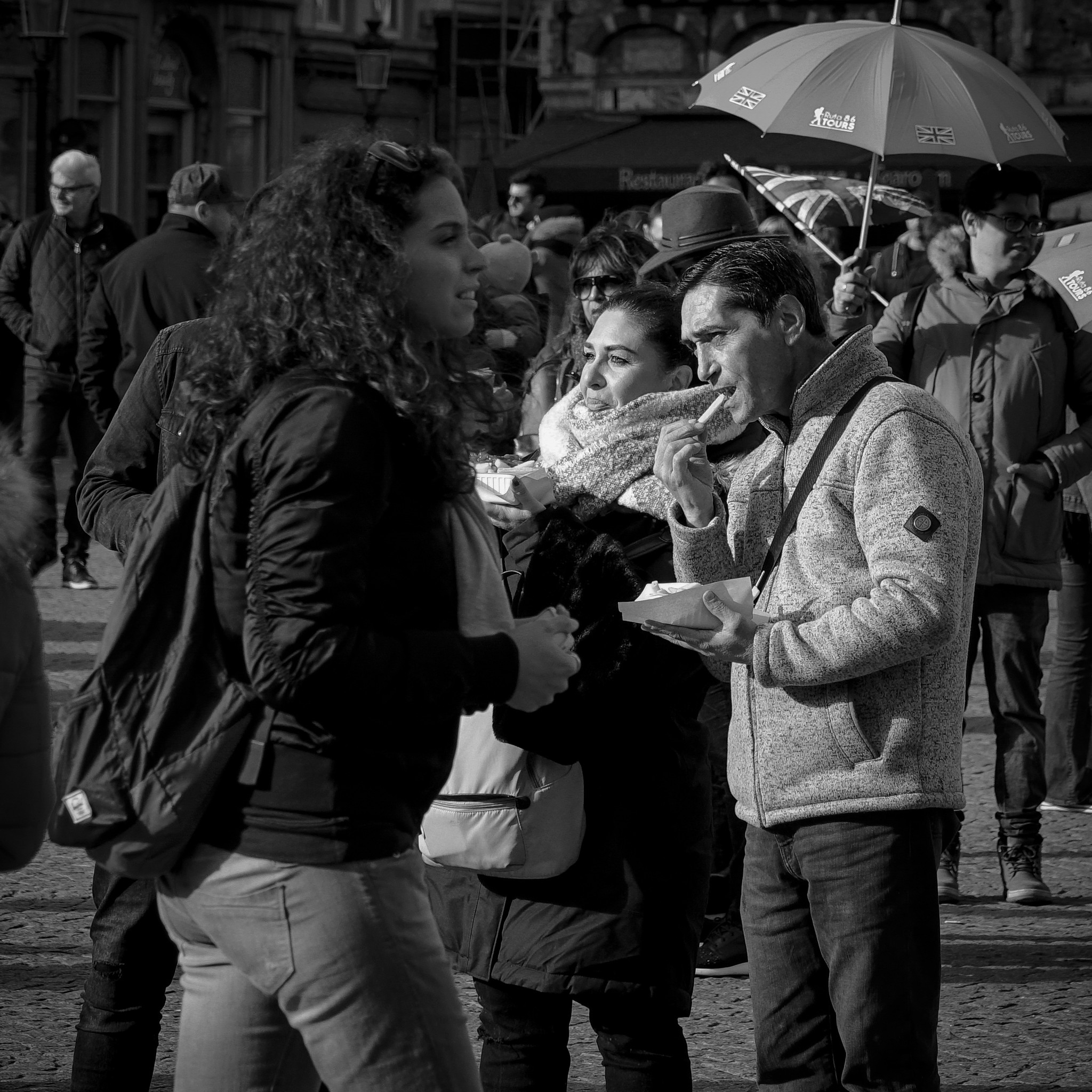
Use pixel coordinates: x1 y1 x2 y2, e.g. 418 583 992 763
937 812 962 900
997 810 1052 901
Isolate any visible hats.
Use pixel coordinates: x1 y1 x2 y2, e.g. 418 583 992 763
478 234 531 293
364 141 452 172
168 162 249 205
638 185 790 276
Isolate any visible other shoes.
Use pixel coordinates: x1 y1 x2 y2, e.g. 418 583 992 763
1039 770 1091 814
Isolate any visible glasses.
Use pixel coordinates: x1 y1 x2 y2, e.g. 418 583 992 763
981 211 1046 237
571 275 629 300
48 182 92 197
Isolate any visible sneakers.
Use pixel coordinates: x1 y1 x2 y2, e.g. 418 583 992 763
26 551 58 579
62 558 98 589
695 915 750 976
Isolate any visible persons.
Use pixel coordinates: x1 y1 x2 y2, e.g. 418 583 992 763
0 140 1091 1091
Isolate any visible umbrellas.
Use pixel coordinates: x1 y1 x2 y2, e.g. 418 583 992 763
688 0 1071 319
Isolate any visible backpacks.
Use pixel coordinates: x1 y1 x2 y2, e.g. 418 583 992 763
418 703 584 877
48 380 389 880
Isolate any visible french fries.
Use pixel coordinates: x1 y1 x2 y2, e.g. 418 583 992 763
639 581 695 600
469 459 537 475
699 393 728 426
